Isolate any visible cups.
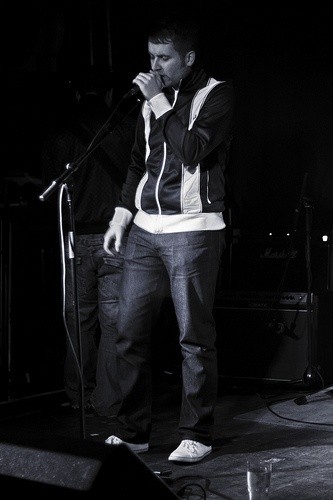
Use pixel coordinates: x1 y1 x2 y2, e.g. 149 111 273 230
246 463 273 500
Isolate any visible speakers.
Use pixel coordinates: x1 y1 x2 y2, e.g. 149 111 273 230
0 429 182 500
207 291 333 391
228 232 333 292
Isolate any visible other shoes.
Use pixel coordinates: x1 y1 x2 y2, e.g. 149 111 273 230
102 435 150 455
83 404 96 416
63 400 79 411
167 438 212 464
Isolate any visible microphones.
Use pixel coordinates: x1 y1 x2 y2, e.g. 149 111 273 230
128 87 140 95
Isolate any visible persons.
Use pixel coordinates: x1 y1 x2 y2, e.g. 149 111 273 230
55 63 128 420
103 23 236 465
